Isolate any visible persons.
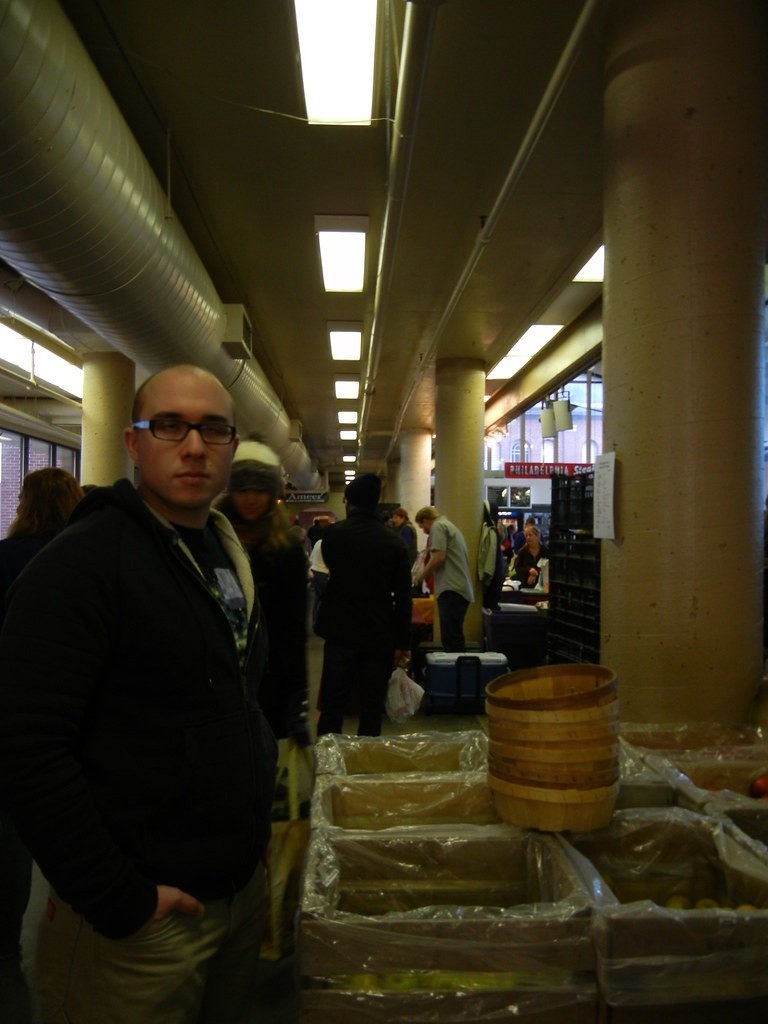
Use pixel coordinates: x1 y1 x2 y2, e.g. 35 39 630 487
0 364 549 1024
392 508 417 561
414 505 474 652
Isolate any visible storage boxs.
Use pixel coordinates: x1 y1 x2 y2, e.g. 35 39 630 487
545 472 600 666
411 581 545 714
299 726 768 1024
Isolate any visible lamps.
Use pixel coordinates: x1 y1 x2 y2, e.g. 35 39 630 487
551 384 573 432
538 395 557 438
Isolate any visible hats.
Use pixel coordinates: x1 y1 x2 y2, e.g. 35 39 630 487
233 441 281 491
345 473 381 508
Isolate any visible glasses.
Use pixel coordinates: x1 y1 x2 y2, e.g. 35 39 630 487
130 418 236 445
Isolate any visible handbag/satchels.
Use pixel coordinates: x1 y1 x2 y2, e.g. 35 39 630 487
385 666 425 724
